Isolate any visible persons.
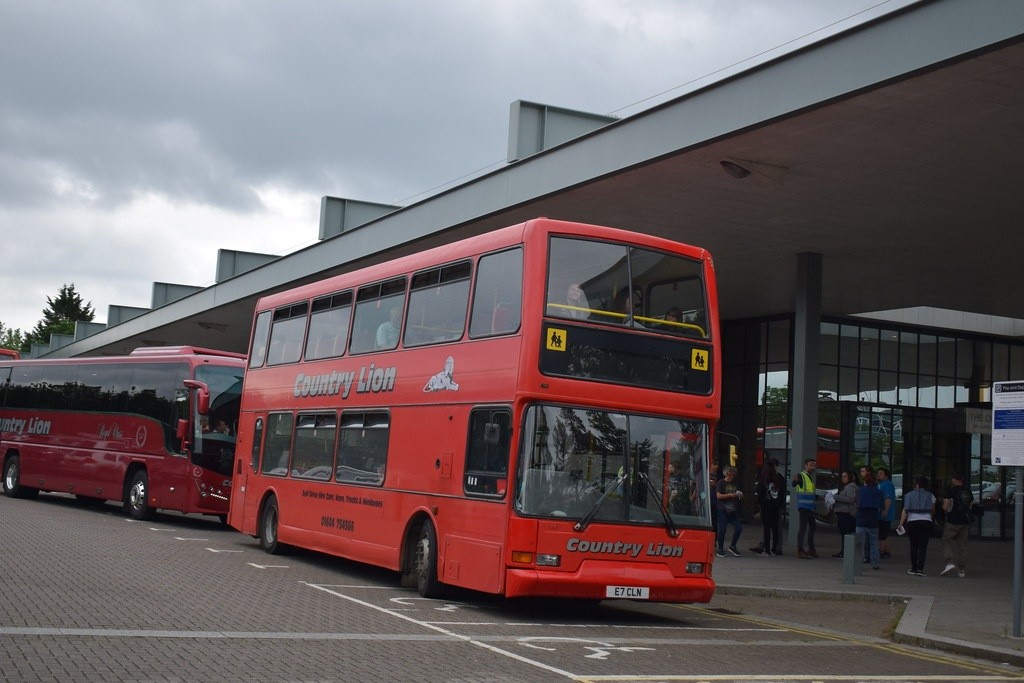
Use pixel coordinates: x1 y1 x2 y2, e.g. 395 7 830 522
215 420 230 435
655 306 683 333
938 473 974 578
668 458 786 557
792 458 896 572
376 304 401 349
603 285 630 324
556 282 590 320
897 476 936 577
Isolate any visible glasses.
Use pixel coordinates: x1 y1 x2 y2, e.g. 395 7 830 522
808 461 817 465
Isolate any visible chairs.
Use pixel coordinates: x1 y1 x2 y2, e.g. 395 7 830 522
269 300 513 363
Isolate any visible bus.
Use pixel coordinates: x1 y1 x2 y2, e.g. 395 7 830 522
758 425 841 470
0 346 251 522
226 215 723 606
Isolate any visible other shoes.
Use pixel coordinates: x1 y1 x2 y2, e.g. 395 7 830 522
797 550 812 558
958 570 965 577
757 551 771 556
940 563 956 575
727 546 741 557
716 551 727 557
832 552 845 559
770 549 783 555
880 551 892 559
907 570 927 577
808 548 818 558
872 568 881 572
